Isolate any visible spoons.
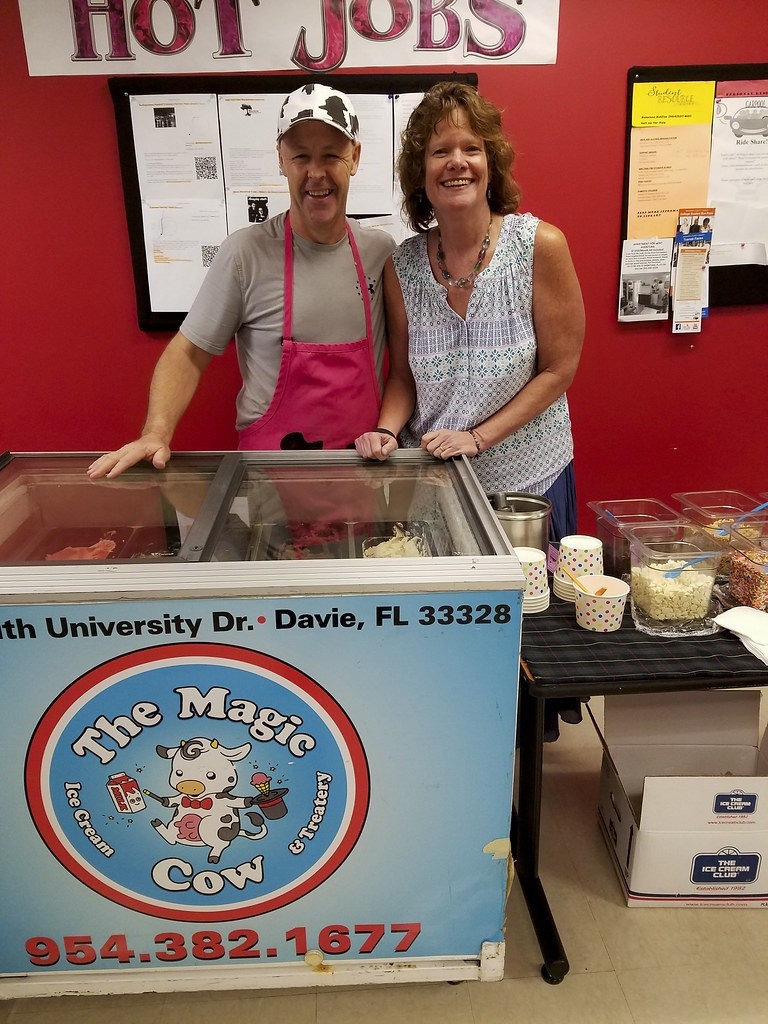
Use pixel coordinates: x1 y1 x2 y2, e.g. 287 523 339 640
662 552 716 578
717 500 768 536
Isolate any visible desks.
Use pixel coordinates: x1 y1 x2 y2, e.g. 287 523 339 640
510 542 768 986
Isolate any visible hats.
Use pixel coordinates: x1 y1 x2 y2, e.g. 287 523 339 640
277 84 360 144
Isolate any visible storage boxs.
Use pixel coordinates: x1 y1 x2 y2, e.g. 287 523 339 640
586 497 691 578
586 690 768 910
670 488 768 574
618 523 735 622
719 522 768 610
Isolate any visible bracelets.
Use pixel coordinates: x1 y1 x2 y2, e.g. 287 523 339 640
468 429 480 456
375 427 397 440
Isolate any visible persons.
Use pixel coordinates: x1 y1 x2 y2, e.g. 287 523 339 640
91 467 451 562
680 218 711 234
353 81 589 747
86 83 396 479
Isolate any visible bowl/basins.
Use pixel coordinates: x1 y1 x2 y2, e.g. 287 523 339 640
513 536 630 632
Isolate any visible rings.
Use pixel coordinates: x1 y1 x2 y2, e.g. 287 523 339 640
439 446 445 452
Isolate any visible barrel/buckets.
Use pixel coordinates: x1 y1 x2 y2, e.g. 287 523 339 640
485 492 553 570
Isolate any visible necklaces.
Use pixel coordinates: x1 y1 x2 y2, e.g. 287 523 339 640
436 208 493 288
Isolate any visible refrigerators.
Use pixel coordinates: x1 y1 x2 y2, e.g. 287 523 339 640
0 445 527 1001
